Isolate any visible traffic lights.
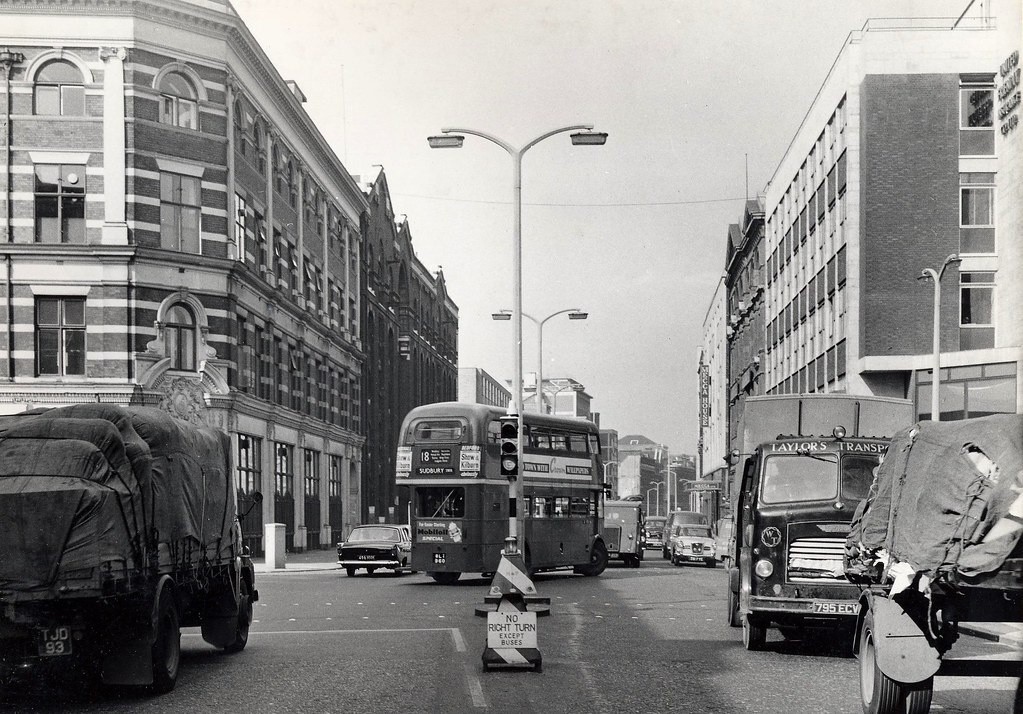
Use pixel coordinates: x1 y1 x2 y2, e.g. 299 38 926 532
500 418 519 476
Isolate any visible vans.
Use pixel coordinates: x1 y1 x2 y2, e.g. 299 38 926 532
663 510 711 560
642 516 667 549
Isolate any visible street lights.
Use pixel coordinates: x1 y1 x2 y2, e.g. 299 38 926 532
651 481 665 516
428 121 608 564
602 461 617 501
492 309 588 414
917 253 962 421
660 469 679 512
527 384 585 415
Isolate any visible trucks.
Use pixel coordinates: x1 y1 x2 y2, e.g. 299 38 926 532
604 500 643 569
845 413 1021 713
1 403 267 697
726 392 914 651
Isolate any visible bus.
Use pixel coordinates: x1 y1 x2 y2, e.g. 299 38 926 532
396 401 608 582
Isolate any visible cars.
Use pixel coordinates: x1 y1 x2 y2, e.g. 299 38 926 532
340 524 416 577
669 523 718 568
